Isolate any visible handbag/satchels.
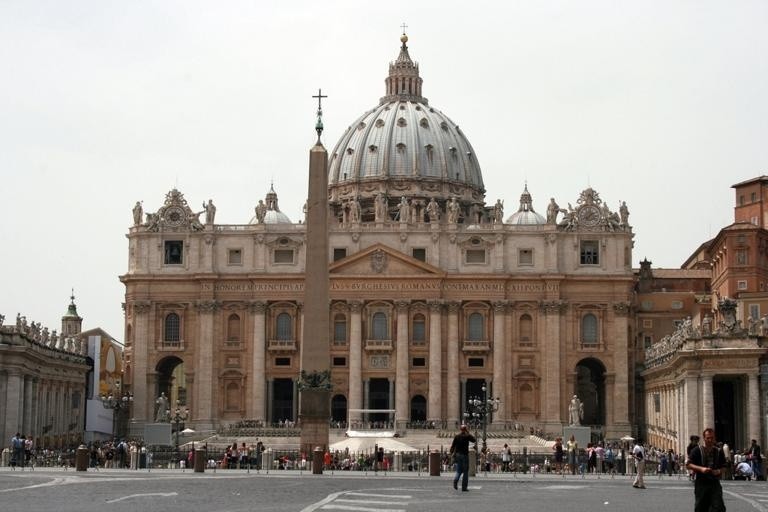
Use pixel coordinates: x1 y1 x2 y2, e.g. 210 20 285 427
637 451 643 462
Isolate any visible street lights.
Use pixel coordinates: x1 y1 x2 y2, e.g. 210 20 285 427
164 399 190 463
100 380 135 439
463 380 501 479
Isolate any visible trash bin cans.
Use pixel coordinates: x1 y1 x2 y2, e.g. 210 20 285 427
75 445 89 471
428 453 440 476
193 449 206 472
313 447 323 474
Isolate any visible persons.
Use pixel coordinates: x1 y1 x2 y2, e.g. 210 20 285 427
448 425 475 492
131 201 145 225
15 312 88 355
201 198 216 225
445 196 461 224
426 195 441 223
396 194 410 222
642 312 766 370
630 438 647 490
345 195 362 224
228 417 434 430
568 394 585 426
373 193 389 222
617 199 630 225
544 196 560 223
683 427 727 510
154 391 171 424
601 201 609 220
254 199 268 224
494 197 504 223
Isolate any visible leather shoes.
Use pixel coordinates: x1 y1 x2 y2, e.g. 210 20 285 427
632 485 645 489
453 479 469 491
